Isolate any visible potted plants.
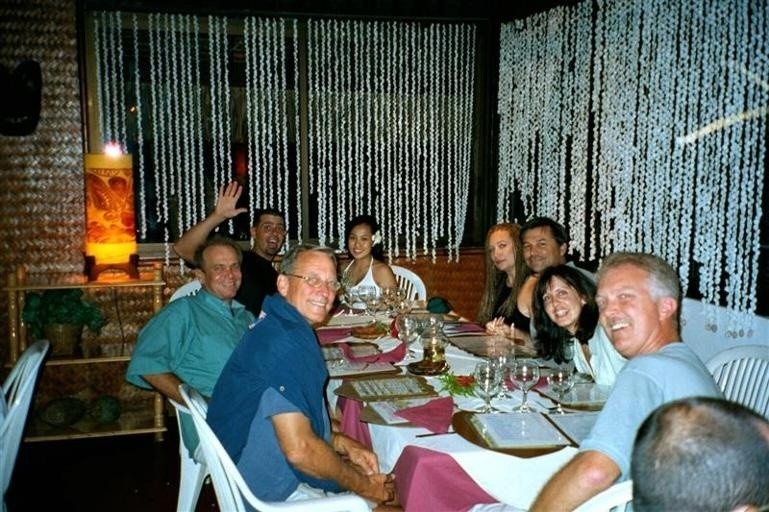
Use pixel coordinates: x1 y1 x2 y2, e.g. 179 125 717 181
21 289 107 357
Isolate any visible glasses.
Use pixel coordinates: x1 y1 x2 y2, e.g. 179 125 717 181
260 224 287 236
282 272 341 290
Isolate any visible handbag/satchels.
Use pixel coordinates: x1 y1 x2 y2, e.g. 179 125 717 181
427 297 455 313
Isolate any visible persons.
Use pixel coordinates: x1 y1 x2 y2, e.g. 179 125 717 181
486 216 597 342
337 215 398 309
124 238 259 467
475 223 537 335
171 180 286 319
629 396 768 512
205 243 407 512
466 251 727 512
531 264 629 386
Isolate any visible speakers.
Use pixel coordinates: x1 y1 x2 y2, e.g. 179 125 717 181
0 52 44 138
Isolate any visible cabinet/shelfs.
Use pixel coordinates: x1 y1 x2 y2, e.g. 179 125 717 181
1 261 168 443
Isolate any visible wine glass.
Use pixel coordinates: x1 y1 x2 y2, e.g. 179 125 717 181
339 281 451 380
473 360 501 414
510 362 539 414
548 372 572 416
489 345 515 400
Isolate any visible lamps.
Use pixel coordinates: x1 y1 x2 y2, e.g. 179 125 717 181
84 154 137 264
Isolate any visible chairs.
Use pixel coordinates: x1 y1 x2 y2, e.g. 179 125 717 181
390 264 427 309
704 346 768 421
0 338 50 512
166 383 370 511
